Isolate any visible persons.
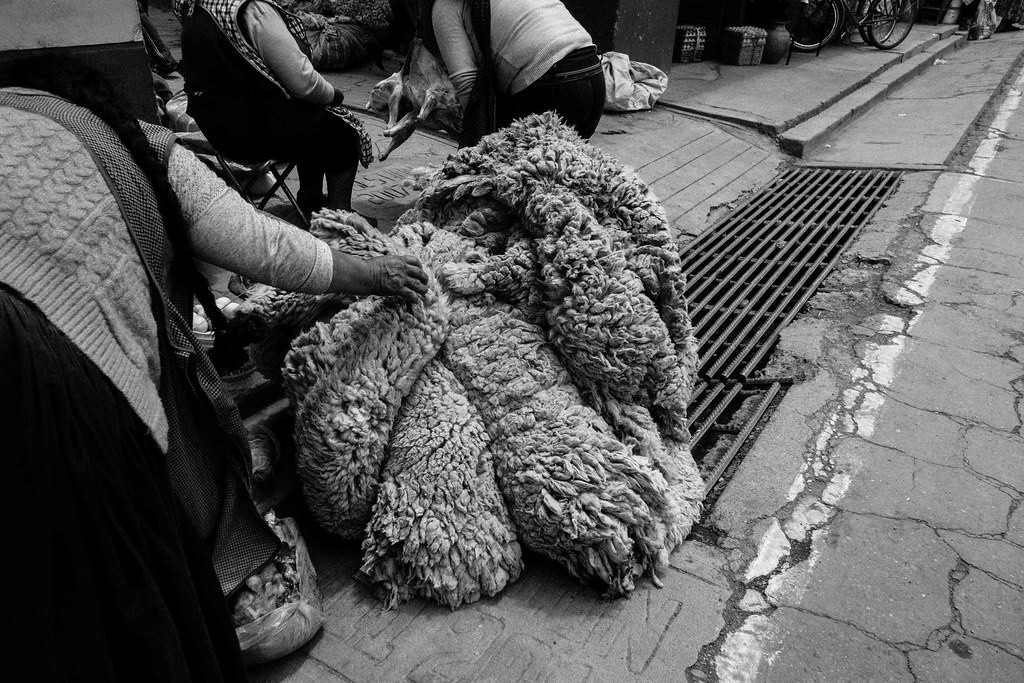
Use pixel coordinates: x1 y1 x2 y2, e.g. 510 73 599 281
0 61 430 682
172 0 379 230
409 0 607 153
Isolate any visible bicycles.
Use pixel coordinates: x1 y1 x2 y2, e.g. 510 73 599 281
786 0 919 54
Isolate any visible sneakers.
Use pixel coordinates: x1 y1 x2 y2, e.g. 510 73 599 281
333 209 379 236
295 192 329 215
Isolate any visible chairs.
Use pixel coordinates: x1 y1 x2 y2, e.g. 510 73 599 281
181 82 316 234
